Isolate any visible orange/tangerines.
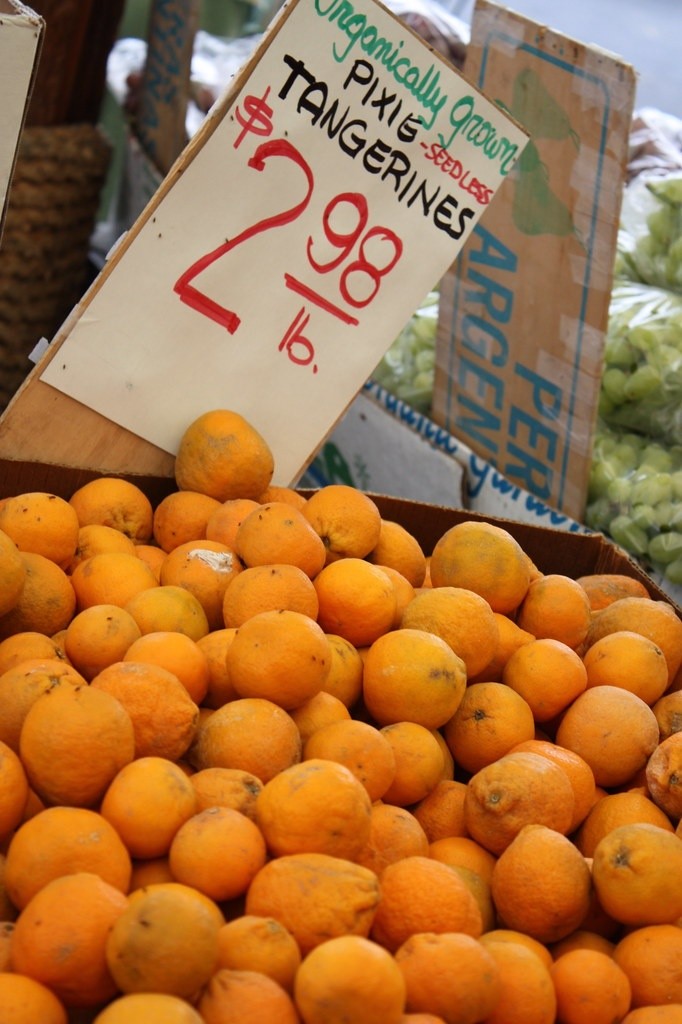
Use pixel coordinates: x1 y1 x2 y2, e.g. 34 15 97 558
0 411 681 1024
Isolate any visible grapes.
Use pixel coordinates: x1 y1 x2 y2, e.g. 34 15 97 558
368 170 682 585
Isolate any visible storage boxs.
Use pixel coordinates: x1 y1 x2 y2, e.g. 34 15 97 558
0 125 118 421
0 374 682 620
